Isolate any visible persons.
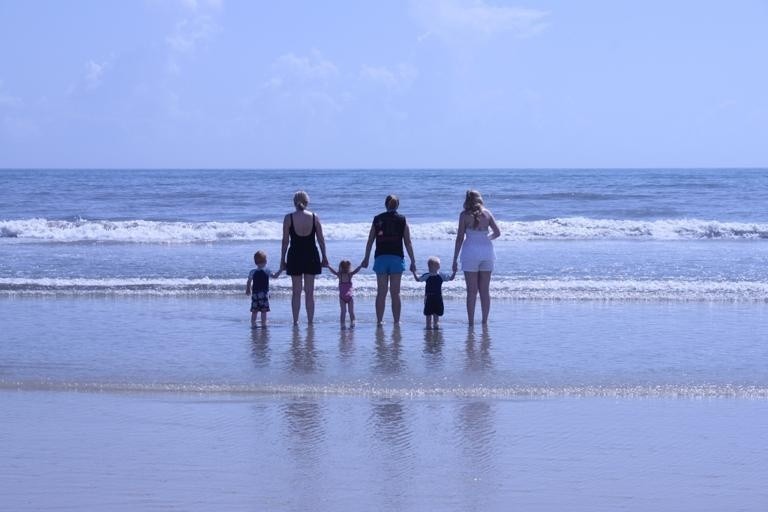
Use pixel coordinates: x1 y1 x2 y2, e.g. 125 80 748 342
452 188 502 326
363 194 417 325
318 262 366 327
409 256 458 330
279 191 329 323
244 250 286 323
